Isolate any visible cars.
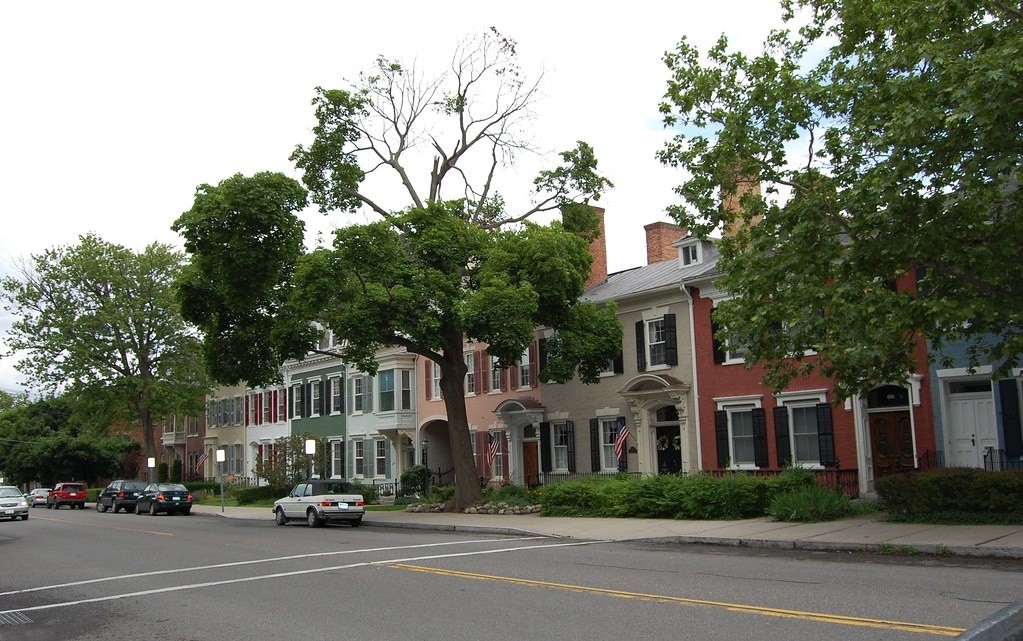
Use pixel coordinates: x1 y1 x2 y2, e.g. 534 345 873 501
0 485 30 521
272 479 365 528
26 488 52 507
133 482 193 516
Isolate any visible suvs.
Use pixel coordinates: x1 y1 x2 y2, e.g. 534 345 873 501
46 481 86 510
95 478 153 513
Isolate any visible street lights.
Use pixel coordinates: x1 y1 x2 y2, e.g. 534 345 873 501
423 435 430 498
147 457 155 484
305 439 315 480
216 449 225 512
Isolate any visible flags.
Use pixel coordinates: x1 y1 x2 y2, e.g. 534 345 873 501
197 449 208 470
487 435 500 467
615 418 630 460
